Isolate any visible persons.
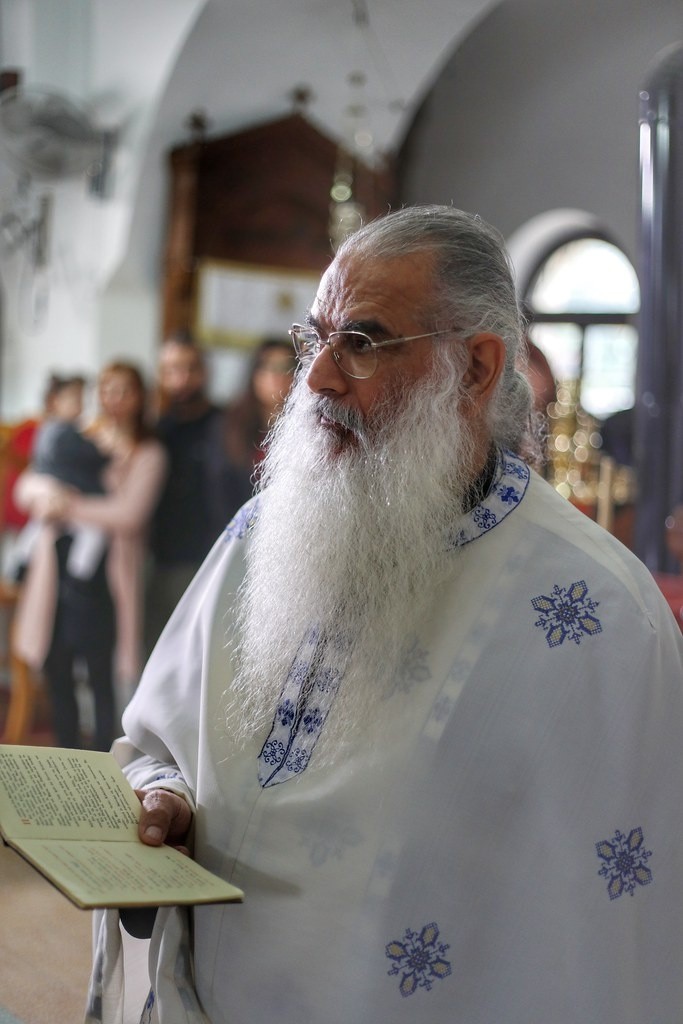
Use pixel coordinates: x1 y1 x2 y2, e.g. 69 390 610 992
0 316 556 753
82 203 682 1024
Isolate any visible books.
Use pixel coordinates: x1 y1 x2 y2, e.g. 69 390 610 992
0 743 245 911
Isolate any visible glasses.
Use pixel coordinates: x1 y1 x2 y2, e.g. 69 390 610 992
286 322 465 382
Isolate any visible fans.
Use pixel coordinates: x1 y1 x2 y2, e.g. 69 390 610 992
0 87 119 198
